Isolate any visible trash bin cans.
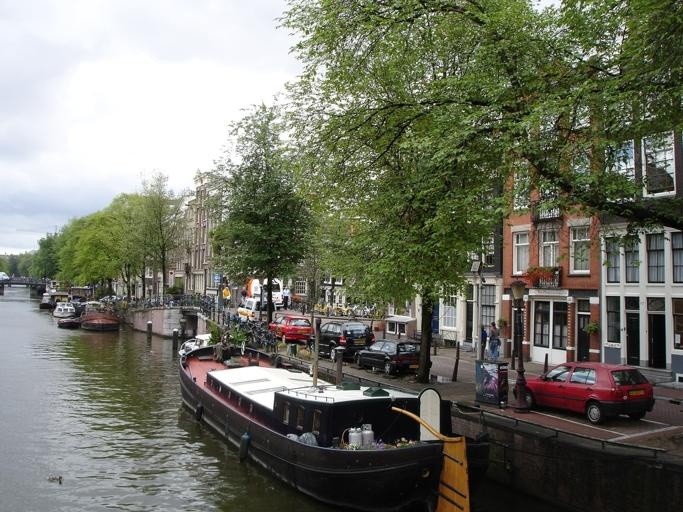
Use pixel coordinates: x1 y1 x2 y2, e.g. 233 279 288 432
474 359 508 408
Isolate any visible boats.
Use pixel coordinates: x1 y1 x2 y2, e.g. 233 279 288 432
179 318 490 512
39 291 119 330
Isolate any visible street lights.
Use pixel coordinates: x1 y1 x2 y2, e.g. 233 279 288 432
511 281 529 414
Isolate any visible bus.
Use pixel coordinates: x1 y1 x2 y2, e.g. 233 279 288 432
250 278 285 305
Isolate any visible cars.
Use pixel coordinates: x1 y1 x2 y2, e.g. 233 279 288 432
513 361 655 424
268 314 432 379
99 295 160 306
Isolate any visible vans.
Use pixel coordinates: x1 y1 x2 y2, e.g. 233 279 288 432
0 272 9 281
237 297 277 324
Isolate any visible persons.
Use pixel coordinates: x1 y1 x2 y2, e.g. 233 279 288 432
489 322 500 361
281 288 289 310
480 324 487 360
241 287 247 297
223 287 231 299
